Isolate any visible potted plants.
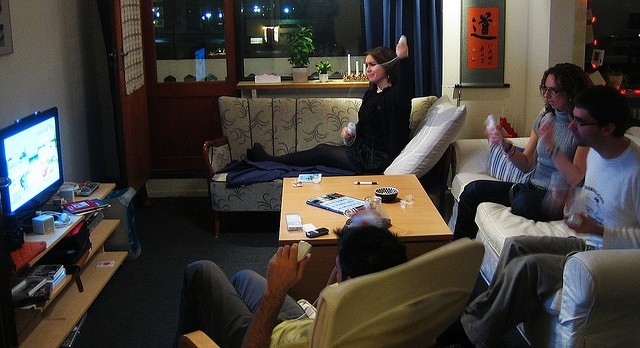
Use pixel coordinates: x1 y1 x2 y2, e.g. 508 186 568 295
315 62 333 82
287 24 316 82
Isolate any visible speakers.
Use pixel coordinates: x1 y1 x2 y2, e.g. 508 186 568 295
59 185 74 204
32 214 56 234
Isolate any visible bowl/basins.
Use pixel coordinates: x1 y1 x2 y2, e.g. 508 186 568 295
374 187 398 201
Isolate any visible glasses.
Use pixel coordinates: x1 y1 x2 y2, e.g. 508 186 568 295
539 85 560 94
567 111 598 128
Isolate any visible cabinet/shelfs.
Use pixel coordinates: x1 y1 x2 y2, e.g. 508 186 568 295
1 182 127 348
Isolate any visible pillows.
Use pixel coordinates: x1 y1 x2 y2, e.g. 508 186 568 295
383 95 467 179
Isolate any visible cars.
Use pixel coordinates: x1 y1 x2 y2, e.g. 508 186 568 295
208 47 226 57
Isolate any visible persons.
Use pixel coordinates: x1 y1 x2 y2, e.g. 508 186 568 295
451 62 599 242
437 83 639 347
172 225 408 348
62 199 99 215
27 264 67 287
306 191 366 216
247 46 411 174
73 199 111 215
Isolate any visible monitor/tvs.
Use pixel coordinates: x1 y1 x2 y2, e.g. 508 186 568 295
0 107 64 228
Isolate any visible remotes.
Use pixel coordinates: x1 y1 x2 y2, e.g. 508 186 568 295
285 215 303 230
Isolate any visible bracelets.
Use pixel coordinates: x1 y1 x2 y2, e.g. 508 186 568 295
504 140 516 157
549 143 561 158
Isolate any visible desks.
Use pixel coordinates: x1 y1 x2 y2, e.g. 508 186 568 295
236 78 371 98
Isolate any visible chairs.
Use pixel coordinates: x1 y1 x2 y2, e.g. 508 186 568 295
179 241 486 348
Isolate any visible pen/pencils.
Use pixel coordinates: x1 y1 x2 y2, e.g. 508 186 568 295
354 181 379 185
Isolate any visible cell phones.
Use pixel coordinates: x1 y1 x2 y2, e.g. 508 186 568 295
306 227 329 237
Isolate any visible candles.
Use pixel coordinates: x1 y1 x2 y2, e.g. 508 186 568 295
355 61 358 75
348 53 351 75
362 63 366 75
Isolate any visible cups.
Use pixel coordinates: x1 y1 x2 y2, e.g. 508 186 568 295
364 197 381 213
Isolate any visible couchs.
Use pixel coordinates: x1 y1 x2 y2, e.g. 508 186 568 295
204 96 453 237
447 127 640 348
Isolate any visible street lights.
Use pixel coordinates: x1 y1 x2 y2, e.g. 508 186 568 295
206 14 212 28
202 16 205 43
